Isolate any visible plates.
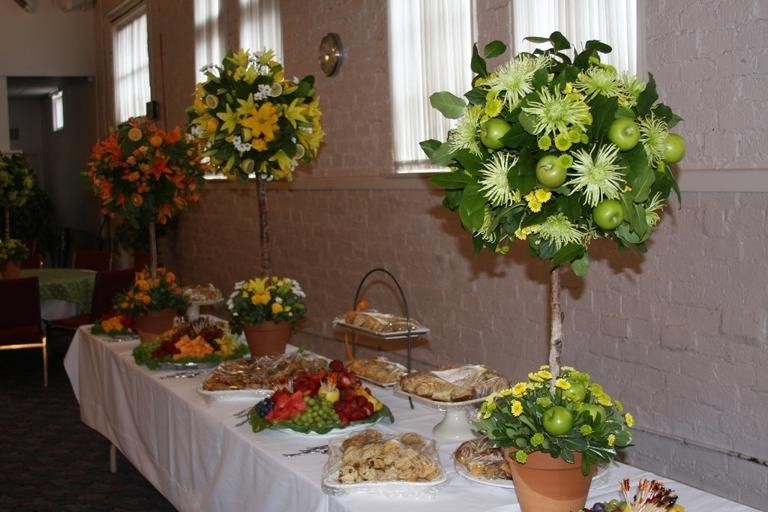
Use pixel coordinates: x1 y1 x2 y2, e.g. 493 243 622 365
354 368 396 391
398 389 489 408
330 316 430 342
322 439 447 489
278 418 385 435
452 451 515 490
194 381 274 403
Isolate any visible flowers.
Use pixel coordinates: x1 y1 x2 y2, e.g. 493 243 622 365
0 152 41 236
420 32 689 387
227 272 307 335
114 264 194 321
78 115 208 277
0 236 33 264
474 359 637 476
183 46 326 270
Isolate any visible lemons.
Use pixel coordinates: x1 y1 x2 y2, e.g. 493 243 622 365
318 388 340 403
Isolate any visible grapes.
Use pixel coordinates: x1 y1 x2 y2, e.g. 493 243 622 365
254 398 274 417
295 396 340 428
594 499 627 512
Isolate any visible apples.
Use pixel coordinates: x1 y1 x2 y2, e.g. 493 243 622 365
662 133 686 163
592 199 624 230
608 119 640 152
535 155 568 188
542 376 606 436
479 118 512 150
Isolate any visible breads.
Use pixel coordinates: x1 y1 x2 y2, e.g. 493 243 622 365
338 429 439 483
456 437 512 481
400 364 509 402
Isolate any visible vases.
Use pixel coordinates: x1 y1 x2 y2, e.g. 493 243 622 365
500 440 596 512
133 308 175 343
242 319 294 356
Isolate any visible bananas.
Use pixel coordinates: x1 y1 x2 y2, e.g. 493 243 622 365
357 390 383 412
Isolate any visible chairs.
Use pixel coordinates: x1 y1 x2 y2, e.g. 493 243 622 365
0 245 152 389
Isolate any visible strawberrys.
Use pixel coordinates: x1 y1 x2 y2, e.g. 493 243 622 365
329 360 374 426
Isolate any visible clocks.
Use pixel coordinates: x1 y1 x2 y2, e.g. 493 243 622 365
318 32 344 78
